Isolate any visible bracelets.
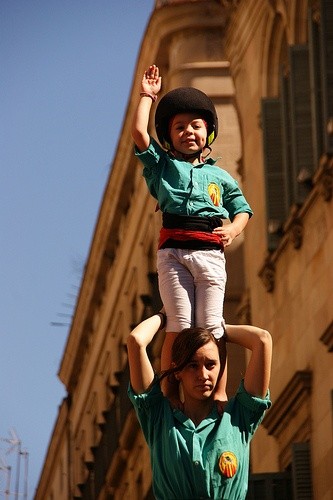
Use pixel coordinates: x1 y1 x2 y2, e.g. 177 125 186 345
140 92 158 103
156 311 166 330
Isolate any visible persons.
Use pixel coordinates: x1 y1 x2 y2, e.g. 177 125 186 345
133 63 253 416
126 305 273 500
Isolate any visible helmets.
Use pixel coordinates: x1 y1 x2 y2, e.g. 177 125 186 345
155 87 218 151
